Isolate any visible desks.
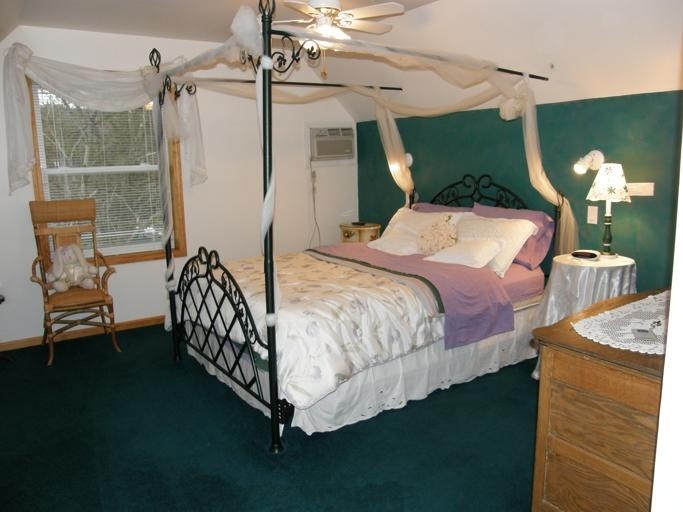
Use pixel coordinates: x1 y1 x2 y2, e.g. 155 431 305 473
534 254 638 381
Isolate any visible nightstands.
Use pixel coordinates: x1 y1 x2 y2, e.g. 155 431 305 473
339 223 379 244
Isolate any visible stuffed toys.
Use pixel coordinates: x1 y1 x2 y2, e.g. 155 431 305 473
45 244 97 293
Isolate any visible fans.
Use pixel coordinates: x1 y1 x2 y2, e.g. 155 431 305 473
271 1 404 35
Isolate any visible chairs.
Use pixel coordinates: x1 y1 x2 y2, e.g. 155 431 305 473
29 197 121 366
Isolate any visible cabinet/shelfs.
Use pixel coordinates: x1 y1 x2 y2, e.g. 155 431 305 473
531 286 670 512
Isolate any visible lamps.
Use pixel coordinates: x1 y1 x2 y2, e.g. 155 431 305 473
299 19 351 78
388 154 413 172
574 150 603 176
587 162 632 254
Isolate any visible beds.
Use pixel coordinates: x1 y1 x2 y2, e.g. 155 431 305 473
149 0 553 453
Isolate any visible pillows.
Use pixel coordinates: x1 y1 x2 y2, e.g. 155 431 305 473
382 204 463 252
412 202 474 213
423 237 502 271
457 212 539 278
472 202 557 270
367 234 419 256
